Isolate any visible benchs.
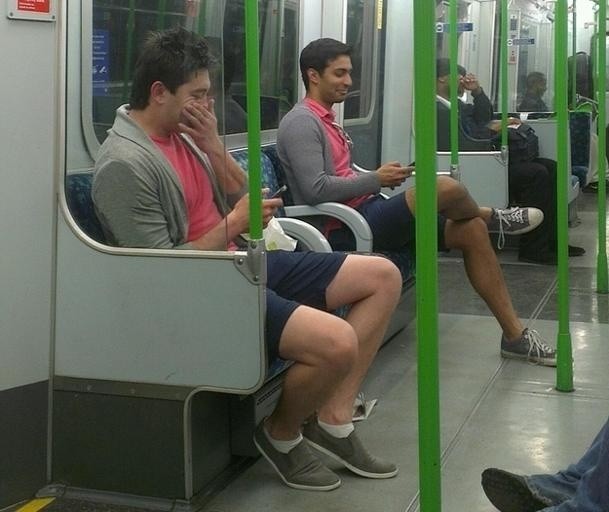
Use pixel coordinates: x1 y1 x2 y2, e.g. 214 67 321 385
47 111 592 512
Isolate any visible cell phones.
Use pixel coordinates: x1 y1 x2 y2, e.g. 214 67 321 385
271 184 288 198
408 161 416 166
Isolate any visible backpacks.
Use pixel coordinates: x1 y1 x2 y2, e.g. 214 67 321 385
488 123 538 163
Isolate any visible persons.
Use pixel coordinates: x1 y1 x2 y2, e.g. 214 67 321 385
480 415 609 511
435 48 608 264
90 25 398 493
274 37 560 368
197 34 259 136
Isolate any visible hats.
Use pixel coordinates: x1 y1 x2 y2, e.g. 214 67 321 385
437 58 466 77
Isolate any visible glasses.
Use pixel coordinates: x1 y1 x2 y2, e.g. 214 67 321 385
332 122 353 149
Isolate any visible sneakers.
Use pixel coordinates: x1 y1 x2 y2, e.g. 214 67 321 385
500 328 557 366
482 468 546 512
487 207 544 234
252 409 398 492
518 236 585 265
